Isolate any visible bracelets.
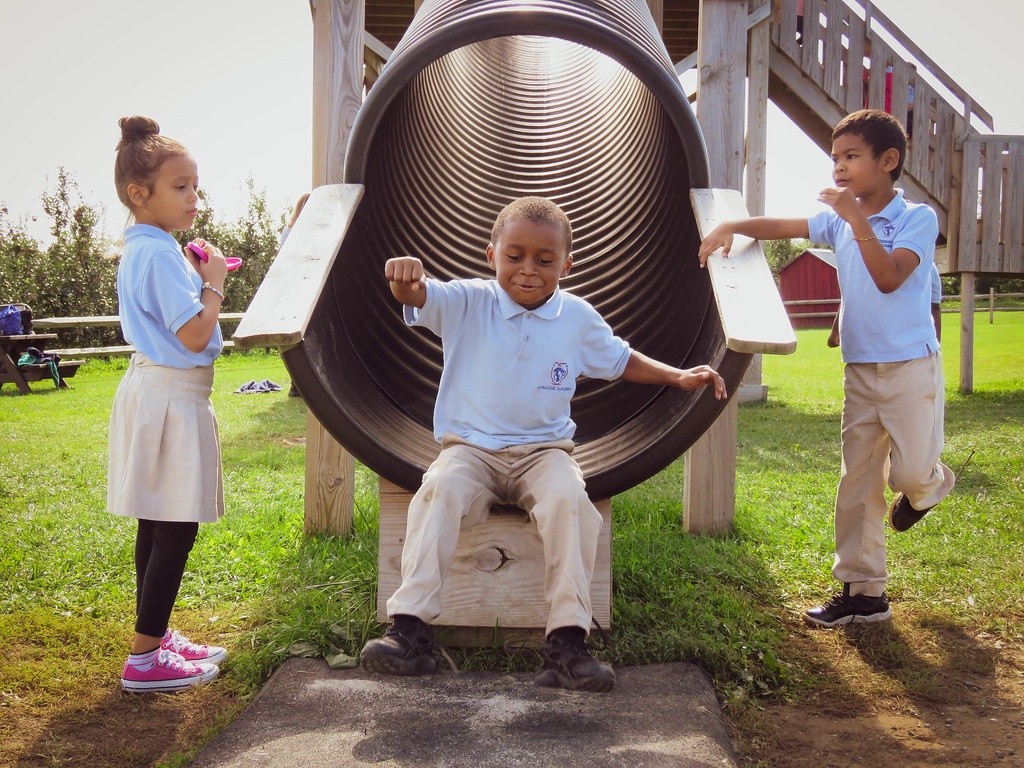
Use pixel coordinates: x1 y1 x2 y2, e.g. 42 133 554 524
201 284 226 303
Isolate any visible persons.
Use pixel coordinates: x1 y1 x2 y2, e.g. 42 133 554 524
360 196 728 693
698 109 957 625
825 256 943 352
104 113 228 694
864 60 926 152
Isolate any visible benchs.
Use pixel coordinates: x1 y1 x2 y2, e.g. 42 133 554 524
19 359 85 379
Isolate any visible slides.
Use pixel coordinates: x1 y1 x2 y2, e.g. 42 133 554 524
240 0 796 507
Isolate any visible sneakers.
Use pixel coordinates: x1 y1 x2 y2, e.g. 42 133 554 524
120 629 227 693
801 591 892 627
533 636 615 691
360 618 439 676
888 493 938 532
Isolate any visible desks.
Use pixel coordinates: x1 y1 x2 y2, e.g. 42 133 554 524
0 332 70 393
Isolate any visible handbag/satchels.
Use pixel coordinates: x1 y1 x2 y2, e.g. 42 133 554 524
0 305 33 335
